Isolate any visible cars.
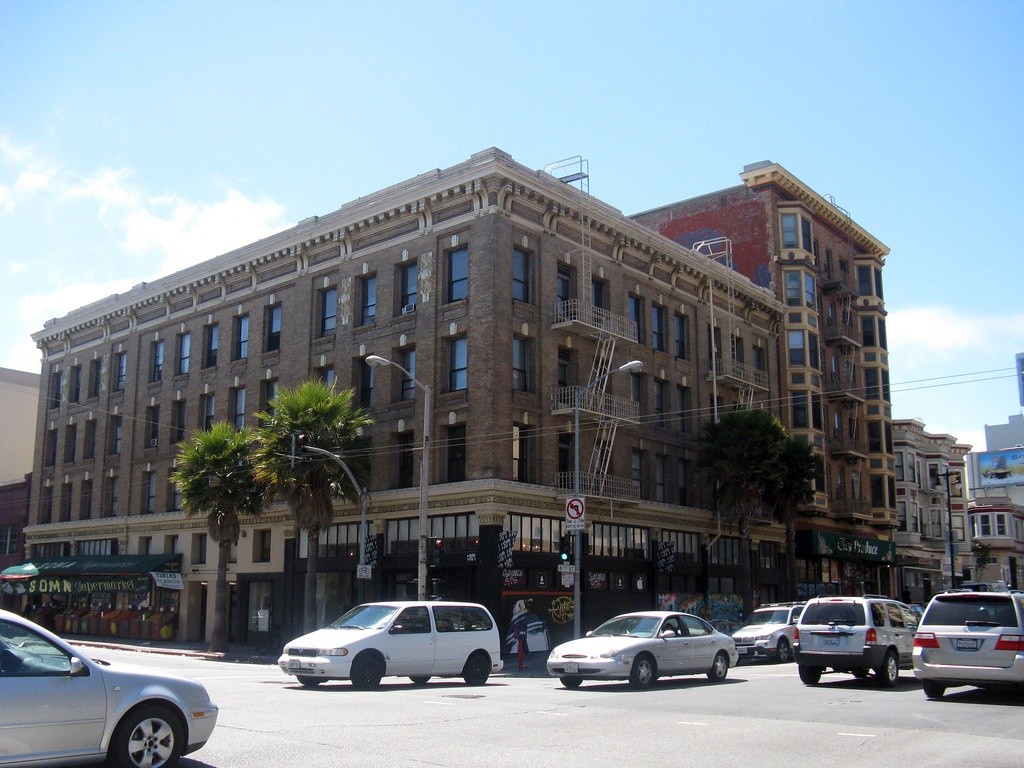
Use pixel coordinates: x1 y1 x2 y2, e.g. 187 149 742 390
0 608 221 768
905 581 1023 620
547 609 740 690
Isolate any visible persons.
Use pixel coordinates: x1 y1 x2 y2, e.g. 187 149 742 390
901 586 912 604
663 624 681 637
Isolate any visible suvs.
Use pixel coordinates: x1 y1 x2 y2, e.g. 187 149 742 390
911 588 1024 701
277 600 504 692
731 601 808 662
792 592 920 689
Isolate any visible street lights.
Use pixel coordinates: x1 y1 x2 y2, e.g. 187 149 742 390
935 465 964 588
364 353 434 618
574 360 645 639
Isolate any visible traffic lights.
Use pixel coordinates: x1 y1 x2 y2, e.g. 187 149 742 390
291 433 309 469
426 536 444 569
431 581 450 596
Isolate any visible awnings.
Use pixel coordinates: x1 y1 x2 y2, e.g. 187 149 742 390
0 552 183 593
